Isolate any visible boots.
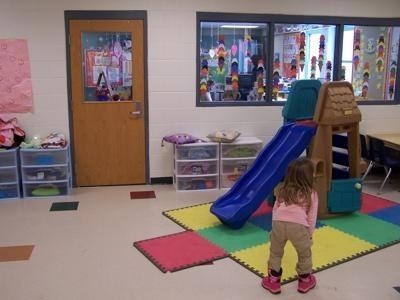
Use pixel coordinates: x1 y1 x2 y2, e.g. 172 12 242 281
261 267 282 294
297 273 316 293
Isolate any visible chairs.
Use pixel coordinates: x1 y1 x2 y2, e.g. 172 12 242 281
360 134 400 195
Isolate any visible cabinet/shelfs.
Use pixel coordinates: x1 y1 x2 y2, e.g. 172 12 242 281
0 147 22 201
171 138 219 192
20 146 72 201
220 137 263 192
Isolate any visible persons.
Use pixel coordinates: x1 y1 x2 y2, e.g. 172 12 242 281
261 158 319 294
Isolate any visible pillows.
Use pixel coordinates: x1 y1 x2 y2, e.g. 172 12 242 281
206 129 241 143
161 133 208 146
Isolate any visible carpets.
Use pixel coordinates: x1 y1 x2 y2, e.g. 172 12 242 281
130 191 156 199
134 192 400 285
49 202 79 211
0 245 35 262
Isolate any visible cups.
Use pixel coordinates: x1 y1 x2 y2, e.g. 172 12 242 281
32 136 41 149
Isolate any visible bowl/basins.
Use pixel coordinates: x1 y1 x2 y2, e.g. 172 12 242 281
205 131 241 143
